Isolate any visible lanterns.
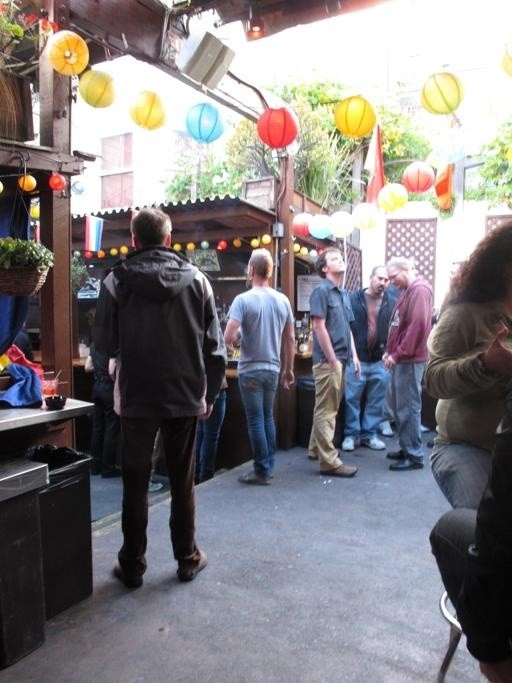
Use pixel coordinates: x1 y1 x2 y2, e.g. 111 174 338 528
79 70 113 109
337 96 377 139
421 71 462 116
256 107 298 148
291 243 322 257
185 105 224 145
173 234 271 254
48 30 89 74
127 93 163 132
291 159 435 238
73 244 129 257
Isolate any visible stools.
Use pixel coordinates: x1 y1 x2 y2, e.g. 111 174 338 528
435 588 475 683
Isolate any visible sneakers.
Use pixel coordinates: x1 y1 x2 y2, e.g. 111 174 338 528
239 469 274 485
341 419 429 451
91 466 164 492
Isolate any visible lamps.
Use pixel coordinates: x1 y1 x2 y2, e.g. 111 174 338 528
244 0 265 39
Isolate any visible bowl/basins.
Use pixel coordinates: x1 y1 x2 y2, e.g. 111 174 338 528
45 395 67 410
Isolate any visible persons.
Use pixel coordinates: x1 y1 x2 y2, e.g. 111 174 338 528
429 404 512 683
307 247 362 477
77 308 228 486
340 265 388 451
421 218 512 510
97 207 209 587
381 255 466 471
223 241 296 485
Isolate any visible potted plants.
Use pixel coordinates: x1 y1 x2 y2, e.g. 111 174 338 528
0 235 56 297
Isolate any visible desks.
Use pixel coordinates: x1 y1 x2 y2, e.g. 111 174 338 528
0 394 95 672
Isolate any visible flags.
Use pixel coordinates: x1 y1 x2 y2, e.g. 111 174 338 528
84 214 104 252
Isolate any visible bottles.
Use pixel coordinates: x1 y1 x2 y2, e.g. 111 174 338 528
78 334 89 360
293 312 313 353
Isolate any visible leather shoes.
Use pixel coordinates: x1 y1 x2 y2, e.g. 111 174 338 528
112 564 143 589
176 549 207 583
319 464 358 477
308 447 341 462
386 448 406 459
389 455 424 471
